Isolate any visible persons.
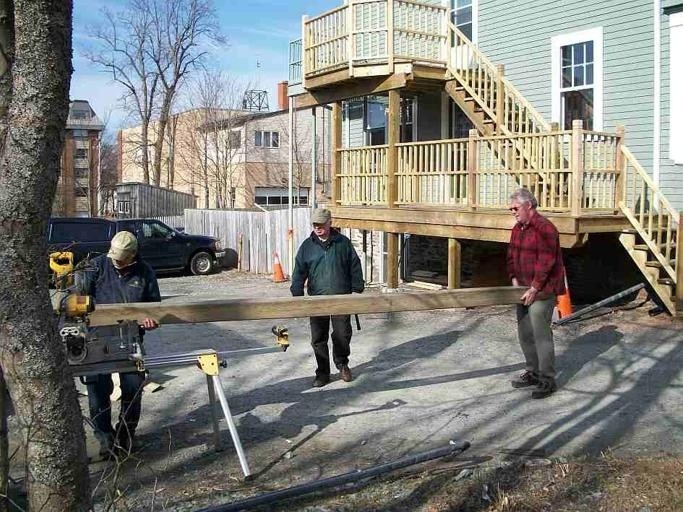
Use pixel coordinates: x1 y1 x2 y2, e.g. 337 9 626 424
288 206 366 387
71 230 161 462
506 188 565 399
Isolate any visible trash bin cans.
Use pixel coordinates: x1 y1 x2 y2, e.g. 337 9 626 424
50 252 76 290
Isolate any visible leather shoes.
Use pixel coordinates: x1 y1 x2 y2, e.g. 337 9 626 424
313 375 330 387
337 364 353 382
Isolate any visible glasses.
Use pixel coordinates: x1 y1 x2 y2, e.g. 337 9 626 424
509 202 525 212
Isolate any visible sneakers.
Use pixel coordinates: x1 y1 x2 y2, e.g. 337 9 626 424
511 369 559 398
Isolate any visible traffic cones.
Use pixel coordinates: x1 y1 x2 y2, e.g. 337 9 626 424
273 253 287 282
557 266 574 320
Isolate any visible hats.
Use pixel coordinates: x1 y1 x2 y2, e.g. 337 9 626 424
311 207 331 224
107 230 138 264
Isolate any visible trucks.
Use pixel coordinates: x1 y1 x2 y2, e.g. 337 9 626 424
45 216 225 289
